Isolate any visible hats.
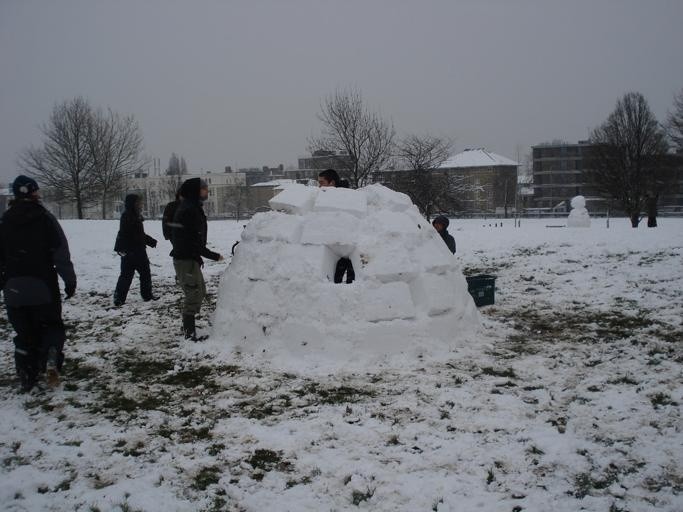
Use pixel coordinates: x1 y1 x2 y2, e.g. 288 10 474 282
13 175 39 195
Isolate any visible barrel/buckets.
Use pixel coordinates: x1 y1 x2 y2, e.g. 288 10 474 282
464 274 497 309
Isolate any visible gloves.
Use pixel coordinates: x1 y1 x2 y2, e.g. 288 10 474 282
64 281 76 300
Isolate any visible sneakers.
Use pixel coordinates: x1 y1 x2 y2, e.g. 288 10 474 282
184 334 201 343
143 295 160 302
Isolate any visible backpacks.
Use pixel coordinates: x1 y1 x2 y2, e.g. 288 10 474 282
162 201 197 240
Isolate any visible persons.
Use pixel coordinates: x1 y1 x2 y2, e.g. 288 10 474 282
432 215 456 255
0 175 76 392
114 194 160 306
318 169 355 284
162 178 225 343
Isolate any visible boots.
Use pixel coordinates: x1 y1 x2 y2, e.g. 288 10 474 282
45 346 59 388
15 349 38 394
